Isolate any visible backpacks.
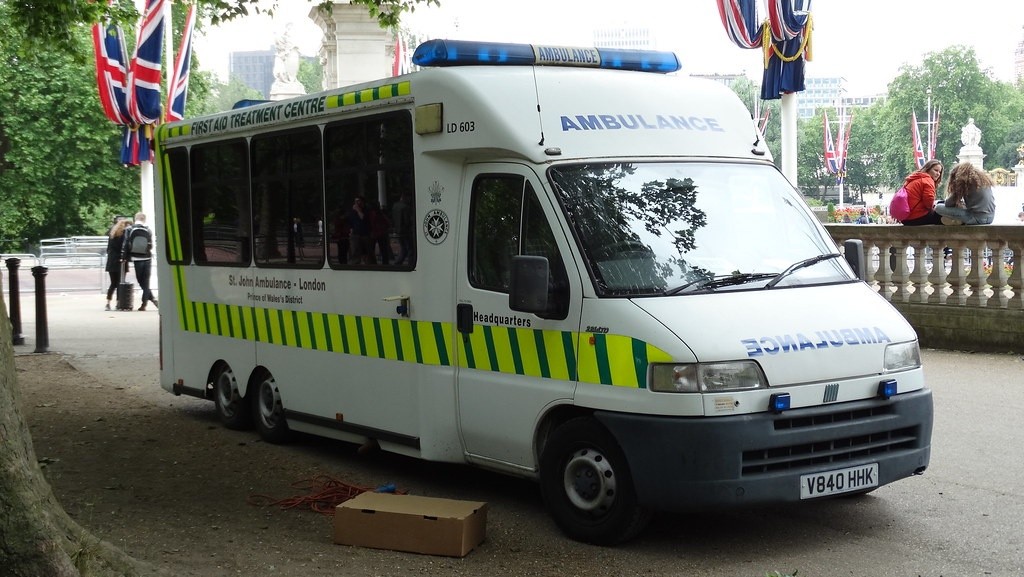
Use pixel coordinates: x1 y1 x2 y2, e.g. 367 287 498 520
855 218 862 224
128 227 150 262
890 186 910 221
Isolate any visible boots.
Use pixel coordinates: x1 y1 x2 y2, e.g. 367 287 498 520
138 301 147 311
152 298 158 308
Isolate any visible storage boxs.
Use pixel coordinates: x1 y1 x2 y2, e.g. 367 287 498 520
332 490 489 559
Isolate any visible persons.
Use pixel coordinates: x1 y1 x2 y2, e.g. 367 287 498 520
334 189 412 266
890 159 944 226
841 213 853 223
105 219 129 311
856 211 868 224
120 213 158 311
934 162 996 226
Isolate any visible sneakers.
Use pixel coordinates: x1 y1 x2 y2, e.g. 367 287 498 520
942 216 962 225
104 304 111 310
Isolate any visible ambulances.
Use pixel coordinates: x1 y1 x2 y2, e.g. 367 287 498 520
152 38 933 550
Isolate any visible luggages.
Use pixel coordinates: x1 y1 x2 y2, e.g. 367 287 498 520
117 261 133 311
